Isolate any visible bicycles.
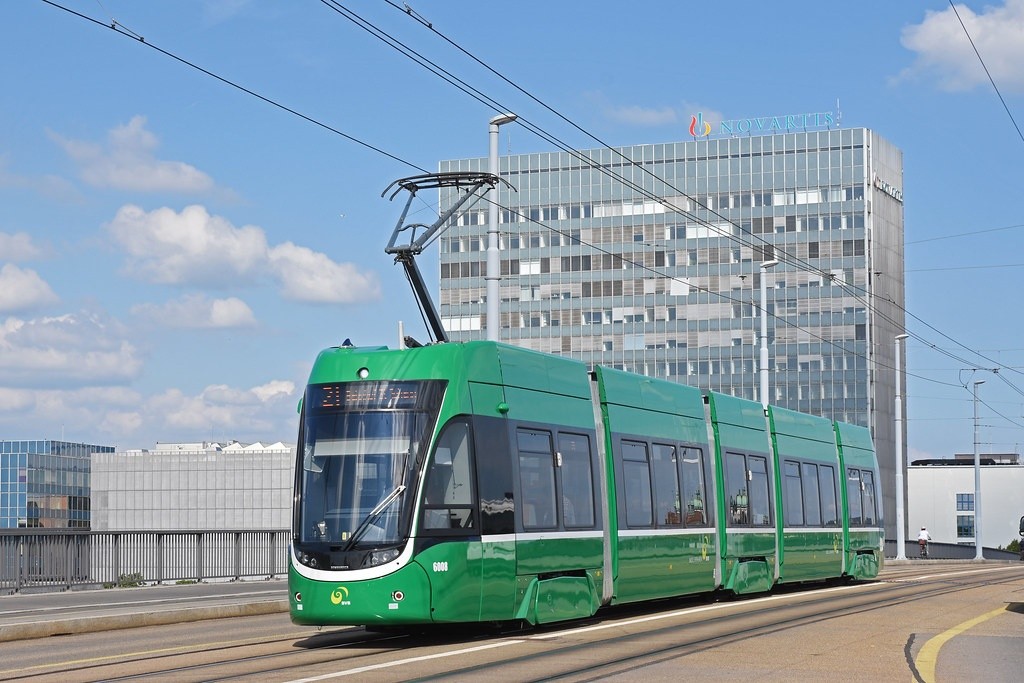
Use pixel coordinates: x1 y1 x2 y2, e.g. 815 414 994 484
919 539 932 559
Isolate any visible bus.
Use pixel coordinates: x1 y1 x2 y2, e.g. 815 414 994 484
287 336 885 638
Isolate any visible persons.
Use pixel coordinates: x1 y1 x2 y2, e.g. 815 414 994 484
918 526 932 556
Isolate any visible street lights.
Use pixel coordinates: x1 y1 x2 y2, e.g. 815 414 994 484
760 259 780 406
485 112 518 342
973 380 986 559
895 333 910 560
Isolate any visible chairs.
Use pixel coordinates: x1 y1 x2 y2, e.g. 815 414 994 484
666 509 681 524
686 509 705 525
